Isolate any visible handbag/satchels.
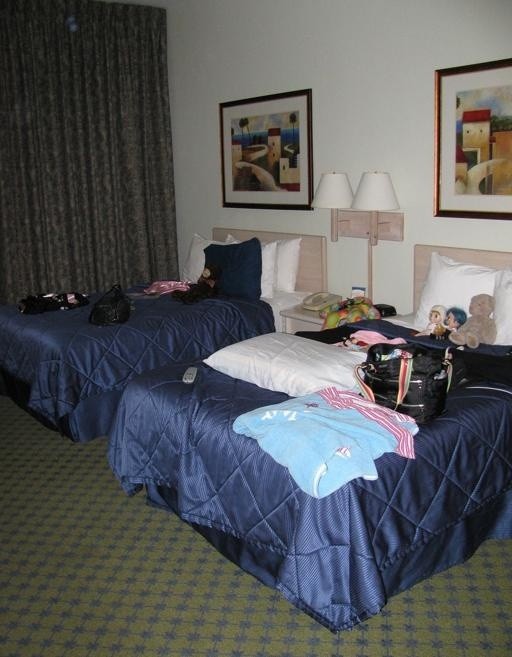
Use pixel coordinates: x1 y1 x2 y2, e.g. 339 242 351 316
88 284 131 326
355 341 470 426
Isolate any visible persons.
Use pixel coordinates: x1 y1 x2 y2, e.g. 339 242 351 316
411 303 446 337
444 306 467 331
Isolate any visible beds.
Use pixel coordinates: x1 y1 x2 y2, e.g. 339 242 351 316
0 225 330 445
106 242 512 636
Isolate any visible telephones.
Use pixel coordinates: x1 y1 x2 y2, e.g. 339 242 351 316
302 292 342 312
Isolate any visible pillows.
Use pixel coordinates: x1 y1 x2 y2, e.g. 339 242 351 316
182 231 241 284
201 330 367 399
491 269 512 347
202 235 262 303
413 250 502 332
225 233 279 301
273 235 302 294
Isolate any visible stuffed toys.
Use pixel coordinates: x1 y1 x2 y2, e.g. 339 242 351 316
171 261 222 305
444 292 498 351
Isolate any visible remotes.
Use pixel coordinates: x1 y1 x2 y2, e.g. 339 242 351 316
182 367 197 384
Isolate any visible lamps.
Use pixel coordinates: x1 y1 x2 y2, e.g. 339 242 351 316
310 169 405 246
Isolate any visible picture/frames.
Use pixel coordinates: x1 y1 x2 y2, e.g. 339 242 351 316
432 57 512 222
217 88 315 213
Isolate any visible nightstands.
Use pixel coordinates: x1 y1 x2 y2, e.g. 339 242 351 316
278 303 326 334
